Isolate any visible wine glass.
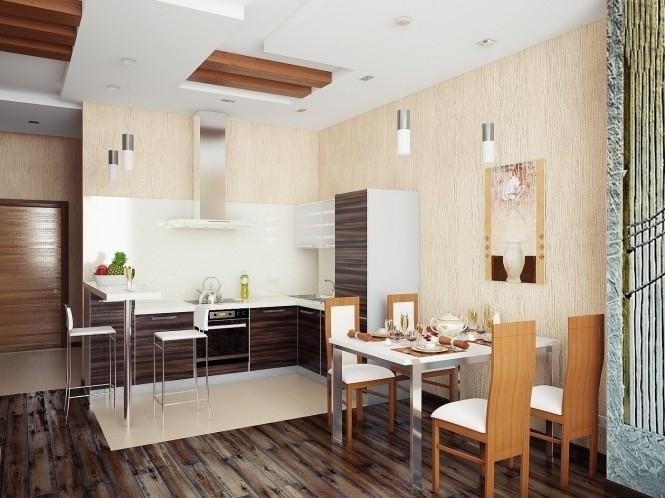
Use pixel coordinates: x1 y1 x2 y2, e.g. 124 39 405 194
125 265 135 291
385 308 500 354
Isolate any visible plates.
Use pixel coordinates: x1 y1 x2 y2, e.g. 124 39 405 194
369 331 389 338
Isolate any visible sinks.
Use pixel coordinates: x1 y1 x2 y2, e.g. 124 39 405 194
287 294 326 299
307 297 324 303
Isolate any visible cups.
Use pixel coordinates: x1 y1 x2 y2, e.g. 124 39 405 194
378 328 387 336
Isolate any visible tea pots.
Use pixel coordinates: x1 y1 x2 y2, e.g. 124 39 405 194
196 276 223 304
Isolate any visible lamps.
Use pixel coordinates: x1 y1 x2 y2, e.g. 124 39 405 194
104 56 137 183
396 17 411 154
480 37 497 163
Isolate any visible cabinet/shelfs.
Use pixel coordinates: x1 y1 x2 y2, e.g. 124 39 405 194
248 305 325 377
335 189 420 395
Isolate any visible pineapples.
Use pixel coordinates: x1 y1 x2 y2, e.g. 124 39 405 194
107 251 126 275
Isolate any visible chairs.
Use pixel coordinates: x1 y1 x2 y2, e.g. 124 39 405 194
64 279 211 420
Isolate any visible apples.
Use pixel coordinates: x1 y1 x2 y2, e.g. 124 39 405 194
96 268 107 275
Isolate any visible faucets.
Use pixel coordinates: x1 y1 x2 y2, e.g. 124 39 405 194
325 280 335 296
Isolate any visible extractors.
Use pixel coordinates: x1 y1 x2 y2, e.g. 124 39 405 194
169 111 257 232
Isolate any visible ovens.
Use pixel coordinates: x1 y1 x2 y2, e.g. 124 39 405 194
193 304 249 368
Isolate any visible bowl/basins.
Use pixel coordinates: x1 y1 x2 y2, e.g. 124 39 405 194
94 275 128 285
